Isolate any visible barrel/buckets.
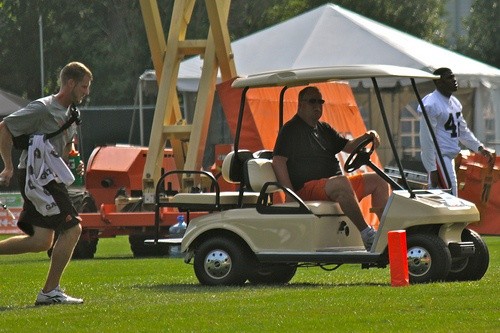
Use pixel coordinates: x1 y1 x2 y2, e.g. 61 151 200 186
168 215 187 257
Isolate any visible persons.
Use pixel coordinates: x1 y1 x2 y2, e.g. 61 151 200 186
0 62 93 306
272 87 392 252
417 66 496 198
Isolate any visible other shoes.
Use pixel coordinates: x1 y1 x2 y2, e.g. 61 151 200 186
363 225 378 251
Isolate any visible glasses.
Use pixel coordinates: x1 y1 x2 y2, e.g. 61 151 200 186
302 98 325 104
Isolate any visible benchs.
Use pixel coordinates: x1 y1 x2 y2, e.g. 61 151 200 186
155 149 274 244
247 157 345 216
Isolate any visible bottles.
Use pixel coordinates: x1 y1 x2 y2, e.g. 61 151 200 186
68 149 84 186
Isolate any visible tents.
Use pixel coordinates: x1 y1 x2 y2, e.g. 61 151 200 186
130 3 500 150
0 89 35 116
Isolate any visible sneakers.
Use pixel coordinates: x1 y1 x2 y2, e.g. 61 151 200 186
36 287 83 307
